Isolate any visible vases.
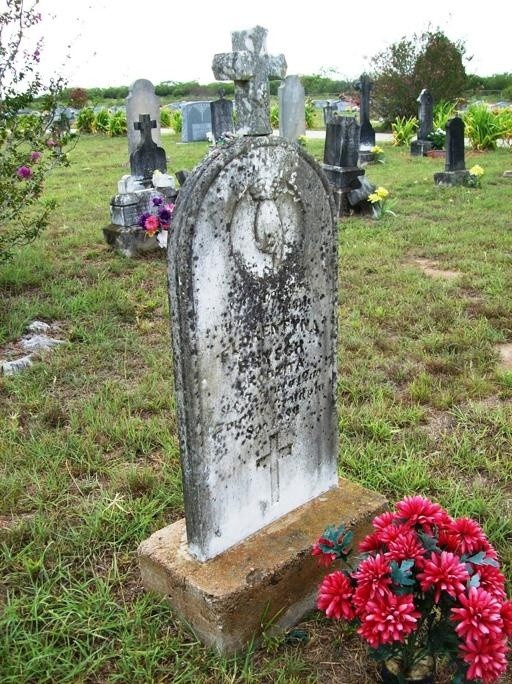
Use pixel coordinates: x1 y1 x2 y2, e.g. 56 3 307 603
381 648 436 683
424 151 446 158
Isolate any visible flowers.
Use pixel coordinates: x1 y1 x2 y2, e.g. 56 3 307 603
311 495 512 684
427 128 446 150
